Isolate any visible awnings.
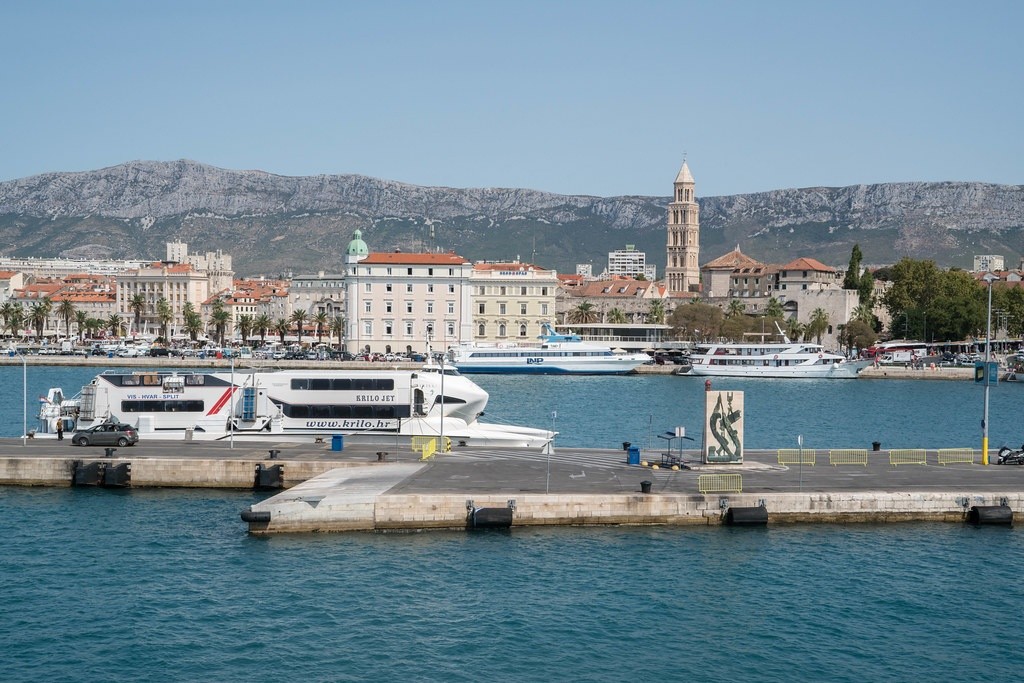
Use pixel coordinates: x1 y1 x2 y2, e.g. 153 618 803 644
657 431 694 470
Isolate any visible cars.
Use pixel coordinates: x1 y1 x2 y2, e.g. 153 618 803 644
72 422 139 447
0 334 426 362
943 350 982 364
1003 349 1024 372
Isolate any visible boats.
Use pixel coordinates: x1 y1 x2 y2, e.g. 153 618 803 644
674 319 876 379
20 330 560 445
446 323 655 375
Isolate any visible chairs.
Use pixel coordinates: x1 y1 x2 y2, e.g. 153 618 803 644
109 427 112 431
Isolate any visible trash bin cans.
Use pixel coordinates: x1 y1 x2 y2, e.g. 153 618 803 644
627 446 640 464
332 435 343 451
872 442 881 451
623 442 631 450
640 481 651 493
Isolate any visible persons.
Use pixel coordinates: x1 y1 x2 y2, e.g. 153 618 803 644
56 418 64 441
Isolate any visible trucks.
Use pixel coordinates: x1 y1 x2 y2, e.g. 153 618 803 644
910 348 927 364
880 351 911 367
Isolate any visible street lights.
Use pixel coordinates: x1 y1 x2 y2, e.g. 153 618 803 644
975 270 1001 464
